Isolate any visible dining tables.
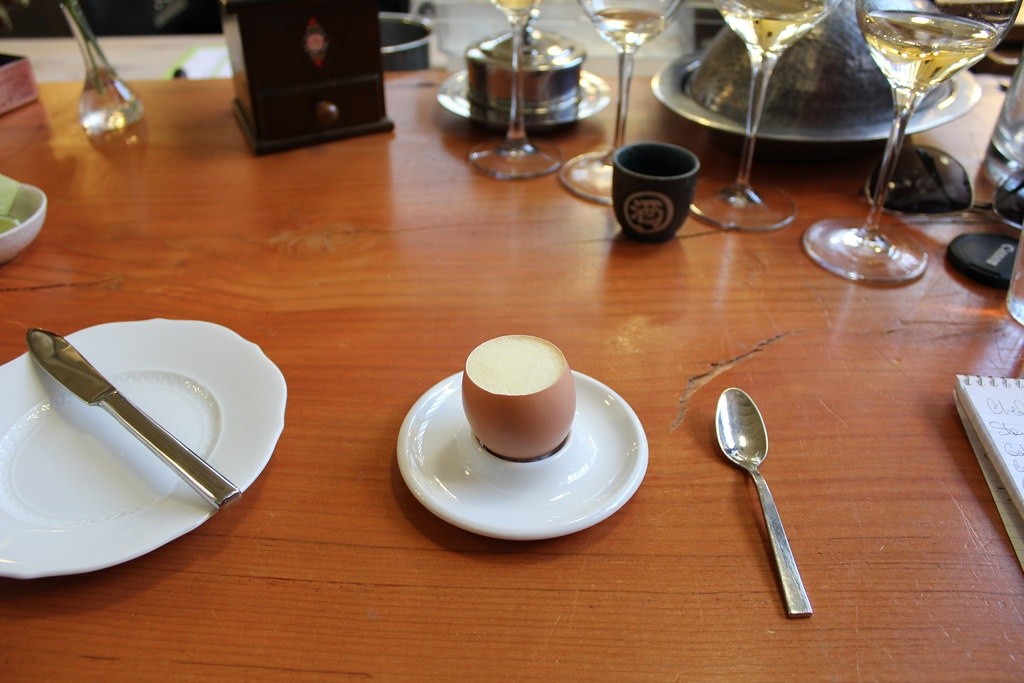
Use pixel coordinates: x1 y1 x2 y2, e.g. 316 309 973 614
1 63 1024 683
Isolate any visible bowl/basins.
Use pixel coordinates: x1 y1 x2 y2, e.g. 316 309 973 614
0 174 48 265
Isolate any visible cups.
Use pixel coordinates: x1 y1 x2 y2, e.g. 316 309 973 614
1007 224 1024 327
974 54 1024 229
377 16 434 74
463 334 573 460
613 144 699 243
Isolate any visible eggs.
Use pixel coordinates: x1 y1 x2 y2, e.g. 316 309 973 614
462 334 576 461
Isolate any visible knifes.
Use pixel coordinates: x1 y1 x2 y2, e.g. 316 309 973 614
25 326 242 512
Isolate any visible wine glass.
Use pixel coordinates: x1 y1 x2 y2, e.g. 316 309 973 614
688 0 842 231
469 0 561 179
560 0 686 202
802 0 1023 286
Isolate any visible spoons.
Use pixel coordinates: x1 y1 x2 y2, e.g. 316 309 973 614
716 387 813 617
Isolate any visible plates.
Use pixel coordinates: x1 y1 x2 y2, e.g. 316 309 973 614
649 49 984 143
438 69 613 127
397 368 648 541
0 318 287 580
